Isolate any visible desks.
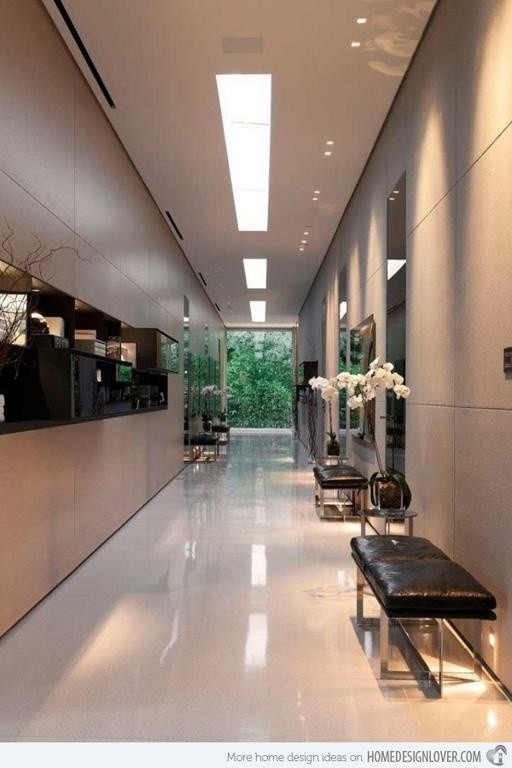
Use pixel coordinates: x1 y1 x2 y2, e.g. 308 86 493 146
358 508 417 537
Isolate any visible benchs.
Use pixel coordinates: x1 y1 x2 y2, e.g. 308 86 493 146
190 434 221 464
213 424 231 445
313 465 368 523
350 534 498 700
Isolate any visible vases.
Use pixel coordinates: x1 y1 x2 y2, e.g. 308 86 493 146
203 421 210 432
372 474 406 517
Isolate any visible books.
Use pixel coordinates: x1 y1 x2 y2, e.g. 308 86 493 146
74 329 107 357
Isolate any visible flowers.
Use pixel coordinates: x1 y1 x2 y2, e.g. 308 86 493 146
199 384 233 423
308 354 412 514
